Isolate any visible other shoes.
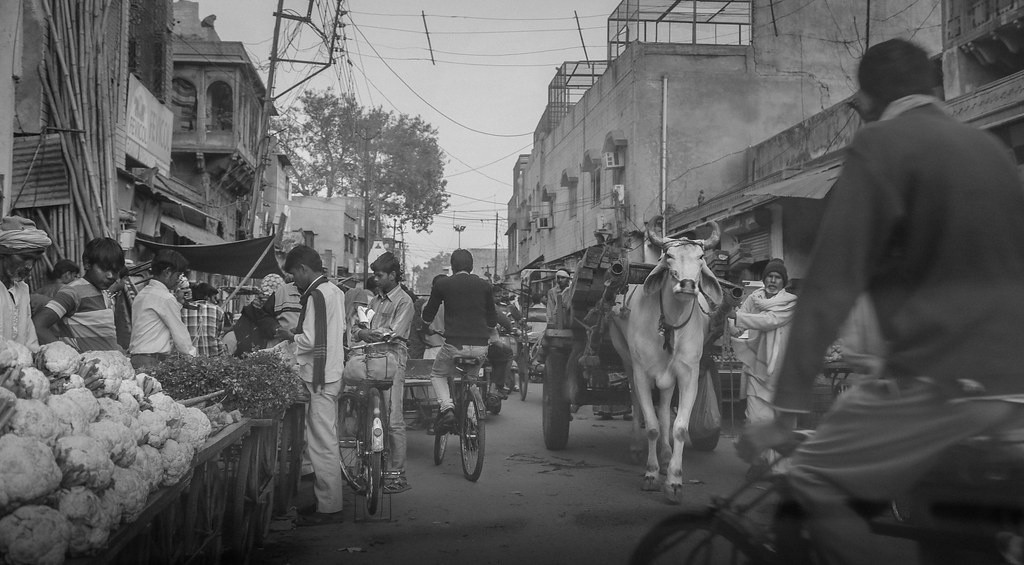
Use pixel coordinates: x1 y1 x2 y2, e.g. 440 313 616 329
293 503 344 526
436 410 456 428
489 390 508 399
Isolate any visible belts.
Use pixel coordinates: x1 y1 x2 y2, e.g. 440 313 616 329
130 354 165 362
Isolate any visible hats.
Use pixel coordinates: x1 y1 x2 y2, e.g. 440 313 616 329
762 258 788 289
0 216 51 250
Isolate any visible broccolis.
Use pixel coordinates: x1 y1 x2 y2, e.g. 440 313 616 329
0 340 212 565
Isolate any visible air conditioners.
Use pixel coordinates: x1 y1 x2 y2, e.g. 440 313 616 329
537 217 553 229
612 184 625 206
528 210 541 223
602 151 624 169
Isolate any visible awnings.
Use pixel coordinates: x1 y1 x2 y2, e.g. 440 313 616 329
134 226 286 314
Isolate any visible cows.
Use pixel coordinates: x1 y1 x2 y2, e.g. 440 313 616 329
606 213 721 504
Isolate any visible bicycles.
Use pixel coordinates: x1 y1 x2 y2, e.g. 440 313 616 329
628 354 1024 565
333 332 413 523
416 326 498 483
494 311 573 402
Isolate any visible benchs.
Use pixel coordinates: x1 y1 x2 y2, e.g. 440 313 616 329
524 311 548 344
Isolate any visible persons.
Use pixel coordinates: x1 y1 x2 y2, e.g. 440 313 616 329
36 259 80 299
235 273 303 362
0 215 52 353
769 40 1024 565
275 245 348 526
347 276 379 358
35 237 125 354
422 249 522 435
728 258 797 456
130 249 197 370
352 252 416 493
180 283 225 358
547 269 570 322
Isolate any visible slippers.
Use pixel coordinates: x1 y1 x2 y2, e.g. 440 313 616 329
384 475 411 492
